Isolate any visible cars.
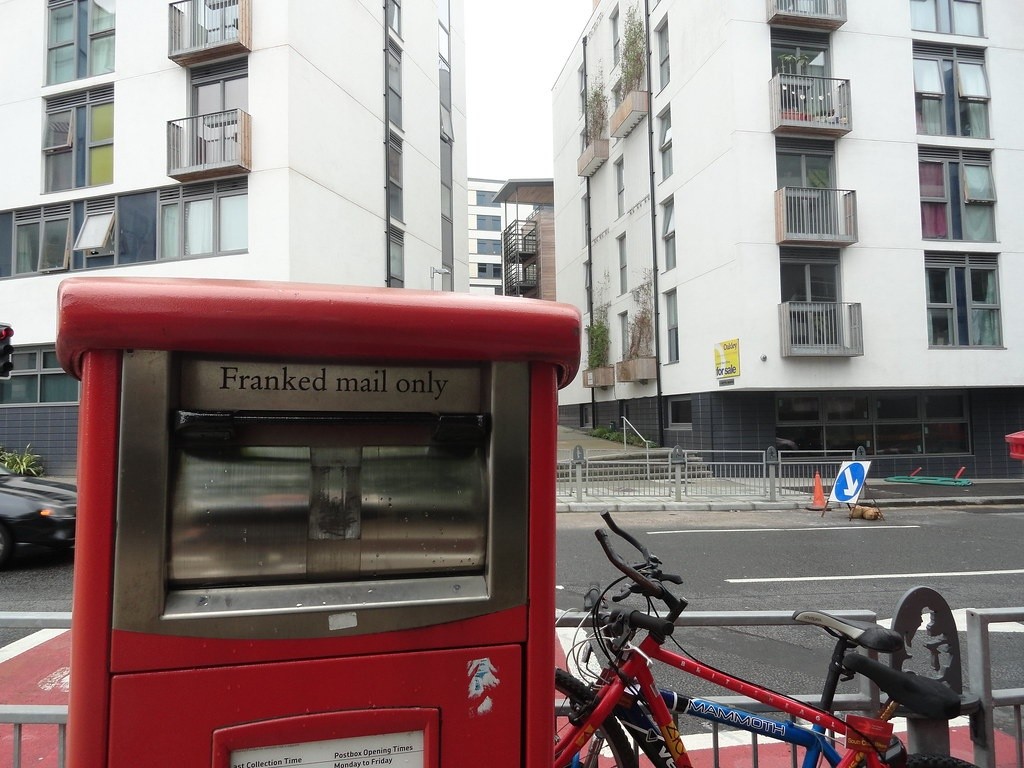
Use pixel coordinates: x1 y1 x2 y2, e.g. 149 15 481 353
0 465 76 566
775 435 923 464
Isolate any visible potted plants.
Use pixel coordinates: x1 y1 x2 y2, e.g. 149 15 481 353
577 81 609 177
616 268 658 382
777 53 814 122
816 111 827 123
608 5 648 137
826 109 836 122
582 317 615 387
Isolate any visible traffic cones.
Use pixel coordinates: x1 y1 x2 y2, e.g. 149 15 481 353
805 470 831 510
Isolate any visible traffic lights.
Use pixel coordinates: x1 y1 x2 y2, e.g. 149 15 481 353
0 322 15 381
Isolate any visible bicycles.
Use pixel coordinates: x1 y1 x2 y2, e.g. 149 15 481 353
552 506 983 767
